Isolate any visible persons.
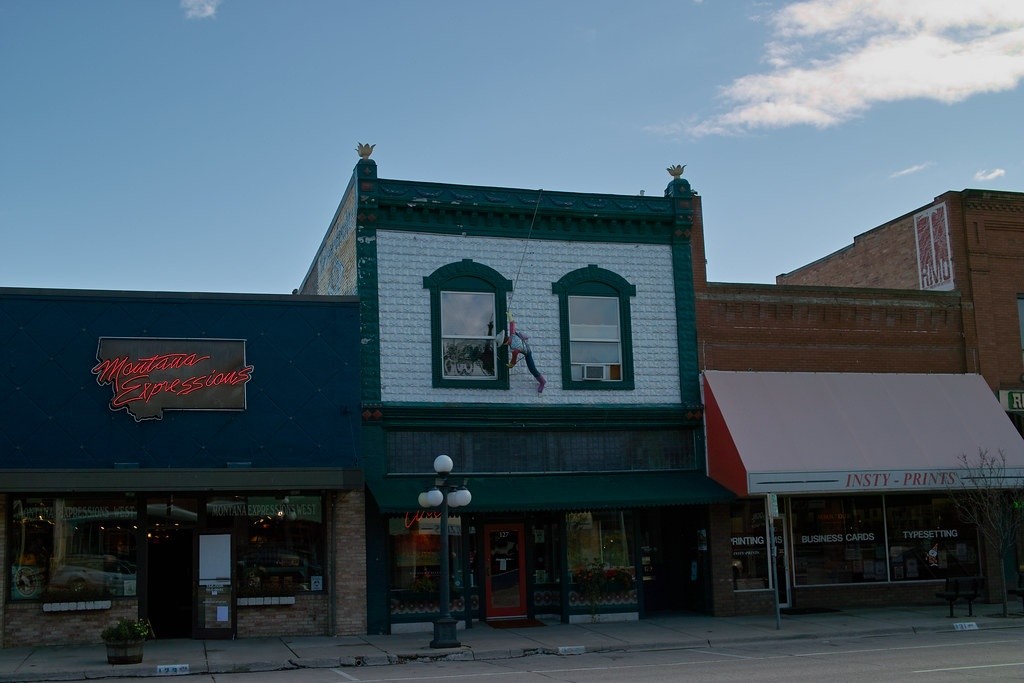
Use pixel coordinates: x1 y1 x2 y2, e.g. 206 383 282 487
497 311 547 394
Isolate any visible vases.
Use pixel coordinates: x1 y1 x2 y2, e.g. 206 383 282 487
576 579 628 597
43 599 111 611
237 596 296 606
106 640 145 664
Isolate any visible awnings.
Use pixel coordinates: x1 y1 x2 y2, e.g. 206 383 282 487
702 372 1024 493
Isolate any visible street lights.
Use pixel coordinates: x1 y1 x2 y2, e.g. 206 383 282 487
418 455 472 649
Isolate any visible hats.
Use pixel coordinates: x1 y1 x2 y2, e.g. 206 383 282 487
495 329 505 348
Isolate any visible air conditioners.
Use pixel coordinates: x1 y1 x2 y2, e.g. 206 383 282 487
583 365 606 380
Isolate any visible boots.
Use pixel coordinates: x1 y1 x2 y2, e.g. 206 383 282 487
534 374 546 393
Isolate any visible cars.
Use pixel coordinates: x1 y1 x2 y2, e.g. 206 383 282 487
236 552 321 591
54 560 136 595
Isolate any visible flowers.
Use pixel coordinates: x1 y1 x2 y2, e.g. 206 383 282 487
575 557 630 585
42 583 112 603
101 617 149 641
238 582 295 598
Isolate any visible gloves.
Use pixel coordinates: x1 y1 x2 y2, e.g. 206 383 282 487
505 311 514 322
505 362 515 368
